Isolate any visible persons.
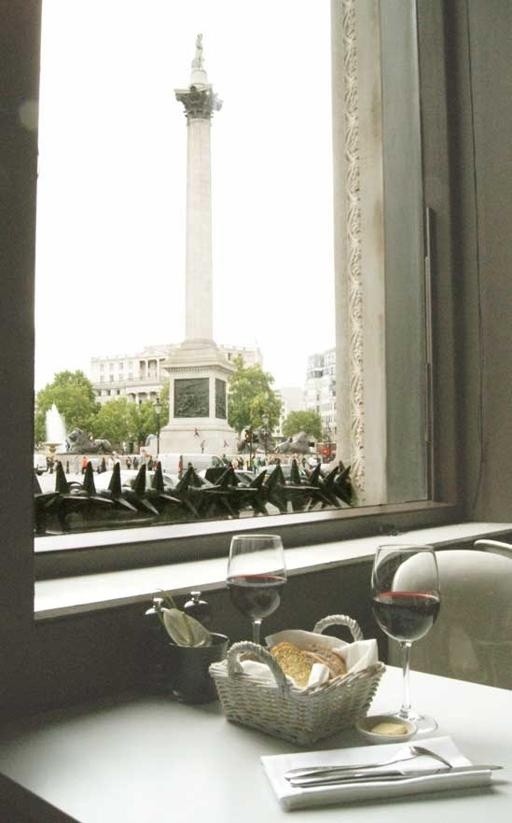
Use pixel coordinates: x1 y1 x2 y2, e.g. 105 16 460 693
45 452 308 480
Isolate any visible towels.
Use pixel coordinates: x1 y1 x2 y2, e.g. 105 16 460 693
260 735 492 812
208 638 378 692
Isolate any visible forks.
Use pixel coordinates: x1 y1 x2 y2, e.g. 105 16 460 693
285 746 453 782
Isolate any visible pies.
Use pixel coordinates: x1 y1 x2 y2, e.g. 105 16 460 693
246 641 347 686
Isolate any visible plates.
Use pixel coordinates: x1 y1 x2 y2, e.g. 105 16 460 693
356 714 417 744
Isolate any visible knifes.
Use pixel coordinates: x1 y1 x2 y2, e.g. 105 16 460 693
291 765 503 786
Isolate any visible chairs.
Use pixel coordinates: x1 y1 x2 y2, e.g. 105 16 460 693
388 549 512 690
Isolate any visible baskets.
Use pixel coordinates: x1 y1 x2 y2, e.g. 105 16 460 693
208 615 386 747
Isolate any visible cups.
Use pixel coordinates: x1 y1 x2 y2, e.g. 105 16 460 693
169 633 230 704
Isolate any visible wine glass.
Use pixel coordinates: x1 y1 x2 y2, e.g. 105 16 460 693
226 535 287 643
371 545 441 735
